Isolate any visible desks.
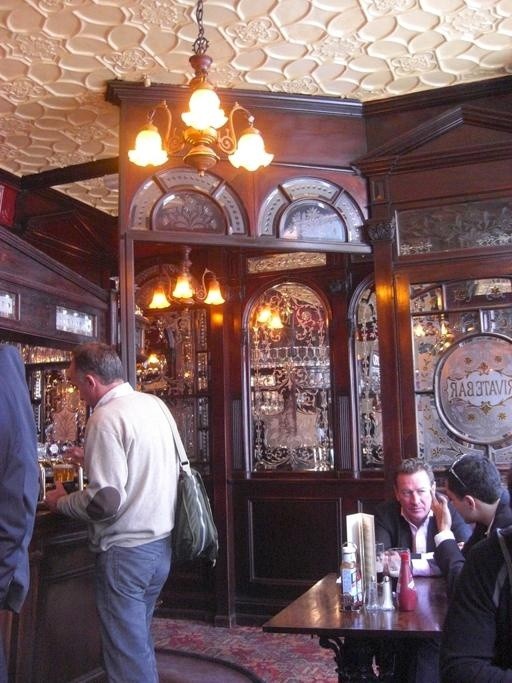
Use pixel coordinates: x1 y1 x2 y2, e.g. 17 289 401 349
262 567 452 682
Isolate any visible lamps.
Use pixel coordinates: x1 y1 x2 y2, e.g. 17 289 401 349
122 1 274 183
145 246 227 312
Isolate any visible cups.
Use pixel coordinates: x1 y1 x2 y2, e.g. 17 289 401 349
386 548 411 578
376 542 385 573
53 463 75 483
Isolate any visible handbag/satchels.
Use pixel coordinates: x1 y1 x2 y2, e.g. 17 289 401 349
173 468 219 576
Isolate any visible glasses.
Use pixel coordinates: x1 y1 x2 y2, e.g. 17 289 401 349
449 454 472 496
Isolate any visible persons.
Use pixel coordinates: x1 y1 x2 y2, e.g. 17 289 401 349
428 456 510 601
42 341 192 683
369 458 473 577
0 340 41 683
445 528 512 681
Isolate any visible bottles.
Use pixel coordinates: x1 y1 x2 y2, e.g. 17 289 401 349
340 546 362 610
366 574 395 613
396 551 418 612
